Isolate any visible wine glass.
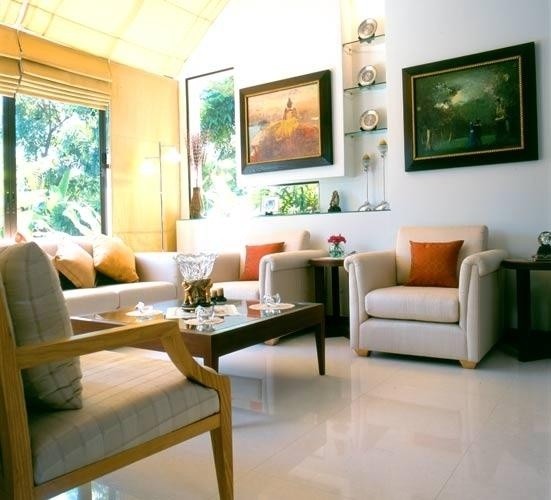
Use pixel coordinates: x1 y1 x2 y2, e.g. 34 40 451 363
171 253 217 311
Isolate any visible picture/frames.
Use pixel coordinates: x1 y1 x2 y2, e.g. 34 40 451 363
239 68 335 176
402 42 540 171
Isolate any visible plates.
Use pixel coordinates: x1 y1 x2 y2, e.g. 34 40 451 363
358 108 380 131
356 65 377 86
356 17 378 40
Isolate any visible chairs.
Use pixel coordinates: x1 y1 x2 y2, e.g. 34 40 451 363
0 273 235 500
209 230 327 347
344 223 512 368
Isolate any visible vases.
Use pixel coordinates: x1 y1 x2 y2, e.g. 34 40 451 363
329 243 345 257
191 186 204 219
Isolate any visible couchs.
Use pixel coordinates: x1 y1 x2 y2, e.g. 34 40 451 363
36 236 188 318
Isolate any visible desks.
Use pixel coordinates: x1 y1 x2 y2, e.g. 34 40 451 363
500 257 551 362
308 256 351 339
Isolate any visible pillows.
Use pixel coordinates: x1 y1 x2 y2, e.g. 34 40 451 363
242 242 285 281
92 234 140 282
406 239 465 289
51 237 98 289
1 241 84 409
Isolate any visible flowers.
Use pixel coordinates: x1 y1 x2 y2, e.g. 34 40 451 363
328 233 347 244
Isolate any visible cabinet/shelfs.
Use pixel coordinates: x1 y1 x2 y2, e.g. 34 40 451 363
343 33 388 140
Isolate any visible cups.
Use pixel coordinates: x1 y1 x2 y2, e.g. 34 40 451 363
135 302 152 320
261 292 281 311
194 304 215 330
